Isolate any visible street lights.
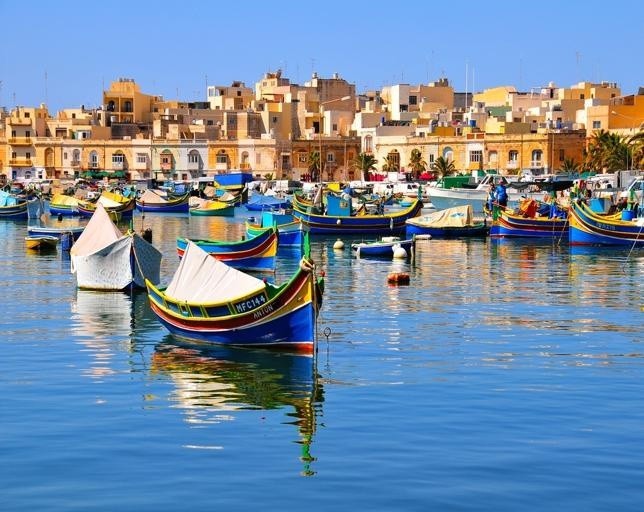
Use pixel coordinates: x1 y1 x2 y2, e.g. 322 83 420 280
610 111 635 170
318 94 352 180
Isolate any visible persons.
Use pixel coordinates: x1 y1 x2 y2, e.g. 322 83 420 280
487 179 509 215
